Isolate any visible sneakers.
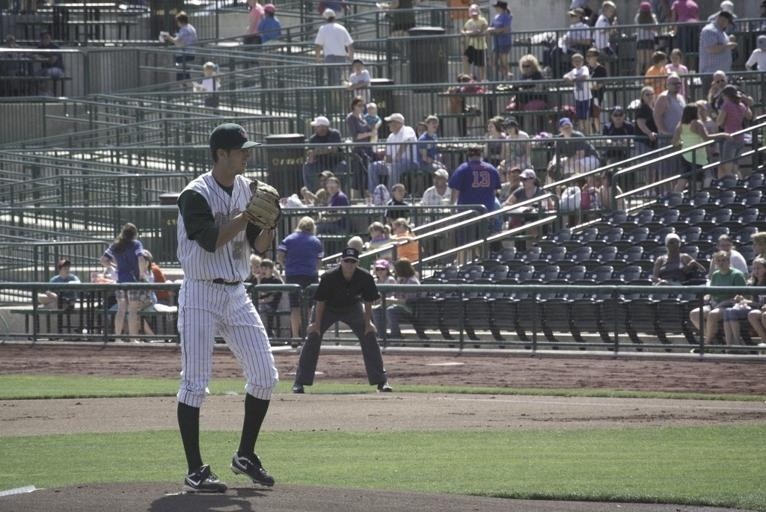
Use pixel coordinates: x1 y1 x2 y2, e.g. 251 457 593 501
292 384 304 393
378 384 392 392
184 464 227 492
230 449 274 487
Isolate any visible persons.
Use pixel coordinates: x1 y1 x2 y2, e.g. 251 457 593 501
37 221 173 348
290 245 394 395
0 0 72 98
143 1 765 356
173 120 280 495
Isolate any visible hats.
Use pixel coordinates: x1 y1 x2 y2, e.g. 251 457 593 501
210 124 262 150
264 4 275 13
58 259 69 269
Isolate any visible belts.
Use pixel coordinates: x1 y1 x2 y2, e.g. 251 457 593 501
213 278 240 285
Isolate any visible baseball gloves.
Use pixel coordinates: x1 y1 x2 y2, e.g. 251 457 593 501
242 178 280 229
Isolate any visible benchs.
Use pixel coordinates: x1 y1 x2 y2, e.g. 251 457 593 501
0 0 151 102
15 270 299 345
